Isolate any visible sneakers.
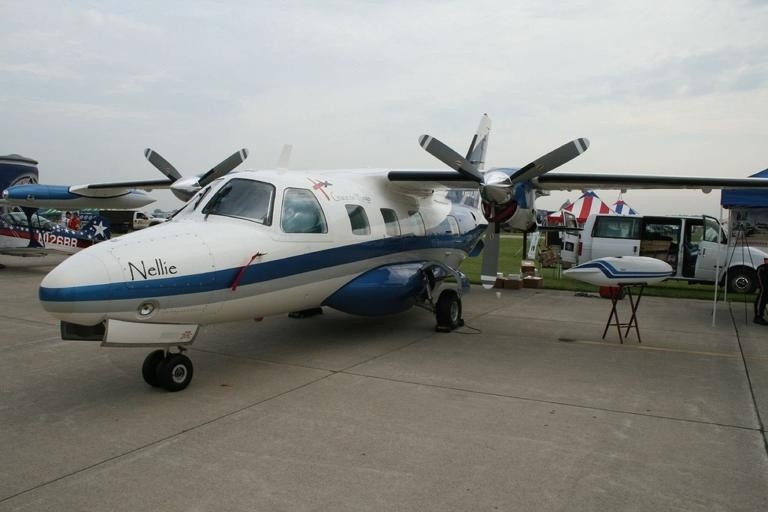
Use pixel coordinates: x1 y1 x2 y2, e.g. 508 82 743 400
754 318 768 325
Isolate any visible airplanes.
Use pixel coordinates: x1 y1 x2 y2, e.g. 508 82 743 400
0 208 113 262
0 109 768 395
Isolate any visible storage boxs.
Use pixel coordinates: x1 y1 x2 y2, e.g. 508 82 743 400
493 259 542 290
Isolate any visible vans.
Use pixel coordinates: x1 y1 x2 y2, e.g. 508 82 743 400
559 205 768 296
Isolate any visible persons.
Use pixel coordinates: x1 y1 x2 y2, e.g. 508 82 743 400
754 257 768 325
63 212 81 229
